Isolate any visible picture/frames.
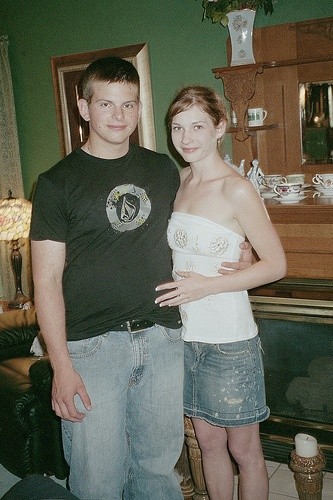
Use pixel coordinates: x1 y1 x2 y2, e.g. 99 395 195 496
50 42 156 160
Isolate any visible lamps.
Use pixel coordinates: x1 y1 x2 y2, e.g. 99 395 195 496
0 190 33 310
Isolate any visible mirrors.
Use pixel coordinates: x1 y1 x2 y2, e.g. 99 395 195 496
297 60 333 172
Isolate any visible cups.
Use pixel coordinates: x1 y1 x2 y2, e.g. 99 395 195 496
261 173 333 198
248 107 267 127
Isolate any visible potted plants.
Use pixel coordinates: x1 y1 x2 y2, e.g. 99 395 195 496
202 0 276 67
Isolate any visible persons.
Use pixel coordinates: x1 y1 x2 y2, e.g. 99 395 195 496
155 86 287 500
30 56 252 499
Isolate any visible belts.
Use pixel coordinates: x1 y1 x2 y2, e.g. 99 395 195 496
109 318 156 334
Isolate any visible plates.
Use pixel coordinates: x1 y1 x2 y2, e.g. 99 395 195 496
261 183 333 204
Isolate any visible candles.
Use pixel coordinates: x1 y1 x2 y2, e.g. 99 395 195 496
295 432 317 457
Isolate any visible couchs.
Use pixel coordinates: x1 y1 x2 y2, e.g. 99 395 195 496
0 309 68 479
